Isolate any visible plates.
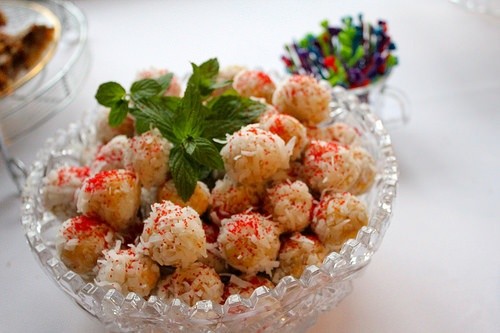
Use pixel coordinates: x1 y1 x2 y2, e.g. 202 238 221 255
0 0 90 146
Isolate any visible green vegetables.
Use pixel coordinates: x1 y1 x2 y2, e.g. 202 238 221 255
95 58 265 203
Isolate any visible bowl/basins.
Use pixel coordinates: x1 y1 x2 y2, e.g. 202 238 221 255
20 64 400 333
